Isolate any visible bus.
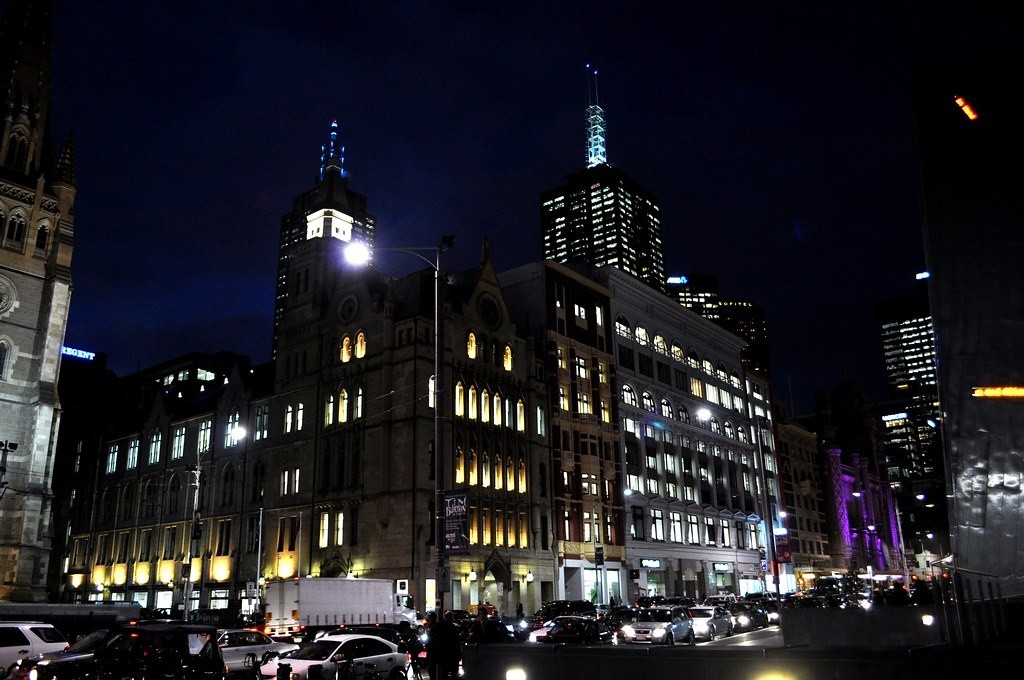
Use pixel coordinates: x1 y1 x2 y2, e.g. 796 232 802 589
95 600 143 608
0 599 142 638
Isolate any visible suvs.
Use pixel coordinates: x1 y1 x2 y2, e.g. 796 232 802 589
3 621 230 680
622 604 697 646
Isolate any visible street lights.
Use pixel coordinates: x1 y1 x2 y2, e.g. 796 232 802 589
180 427 247 620
767 500 792 628
338 233 448 615
65 569 91 604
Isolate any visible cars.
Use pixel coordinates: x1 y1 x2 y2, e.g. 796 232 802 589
0 620 70 676
699 593 737 606
688 604 735 640
144 607 179 619
187 609 219 621
745 585 873 624
258 633 412 680
417 612 429 630
311 625 406 650
728 601 768 634
218 627 301 671
528 614 614 645
529 599 597 626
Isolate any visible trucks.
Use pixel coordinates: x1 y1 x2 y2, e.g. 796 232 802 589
262 576 417 630
